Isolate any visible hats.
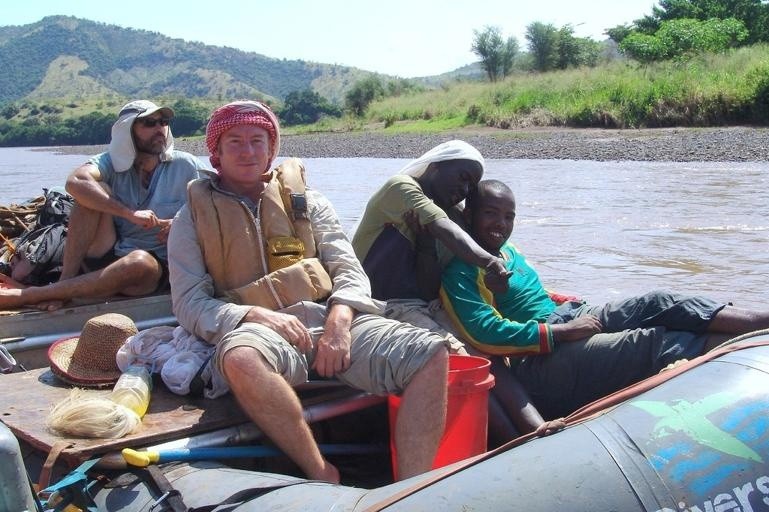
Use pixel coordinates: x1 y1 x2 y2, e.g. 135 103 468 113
110 99 175 173
48 312 142 385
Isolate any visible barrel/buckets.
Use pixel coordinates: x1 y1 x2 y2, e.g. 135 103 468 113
387 354 496 483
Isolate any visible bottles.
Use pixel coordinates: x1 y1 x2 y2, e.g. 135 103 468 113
111 359 153 419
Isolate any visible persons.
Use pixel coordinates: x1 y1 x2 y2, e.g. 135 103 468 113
0 100 208 311
440 180 769 400
352 140 567 445
167 100 450 483
